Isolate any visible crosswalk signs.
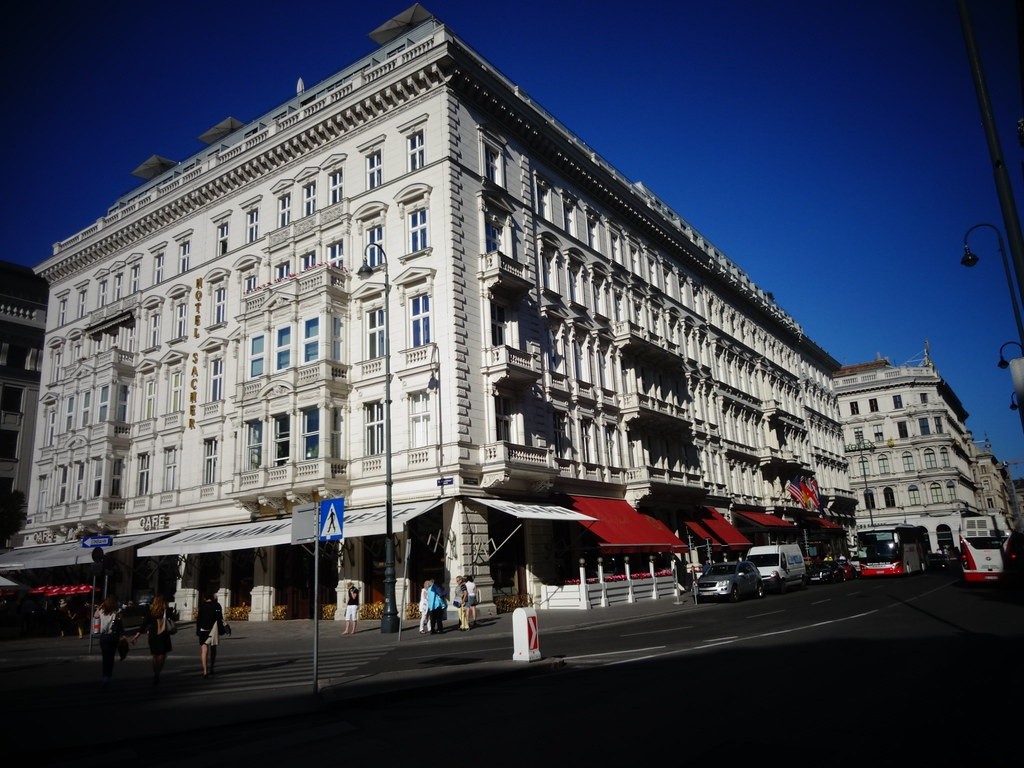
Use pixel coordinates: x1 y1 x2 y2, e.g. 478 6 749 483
319 497 344 541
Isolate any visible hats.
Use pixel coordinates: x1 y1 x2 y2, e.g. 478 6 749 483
346 582 354 589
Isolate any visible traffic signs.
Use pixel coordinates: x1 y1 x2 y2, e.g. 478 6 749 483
81 535 114 548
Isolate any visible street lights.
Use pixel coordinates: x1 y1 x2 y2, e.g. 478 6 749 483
356 243 400 634
960 223 1024 351
859 439 877 527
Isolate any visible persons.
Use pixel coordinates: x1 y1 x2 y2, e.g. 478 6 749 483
419 580 447 634
824 552 851 561
93 595 174 685
196 592 224 679
937 548 950 558
343 582 359 634
55 598 92 639
703 559 710 573
454 576 477 630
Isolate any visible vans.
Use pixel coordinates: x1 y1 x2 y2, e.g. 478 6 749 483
743 543 810 595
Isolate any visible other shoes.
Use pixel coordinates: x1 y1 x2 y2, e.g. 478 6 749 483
342 631 349 634
351 630 355 635
430 629 446 634
420 629 426 634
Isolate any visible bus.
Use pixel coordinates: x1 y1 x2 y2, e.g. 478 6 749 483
852 524 933 579
954 513 1017 584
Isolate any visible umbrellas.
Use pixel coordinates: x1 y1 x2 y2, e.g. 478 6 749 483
32 584 100 596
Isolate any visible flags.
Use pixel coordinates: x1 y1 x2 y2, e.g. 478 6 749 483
787 477 820 510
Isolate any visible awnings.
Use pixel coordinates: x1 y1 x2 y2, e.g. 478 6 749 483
676 504 753 551
790 513 842 528
468 497 599 559
0 532 173 570
731 509 796 528
557 495 689 553
137 499 453 557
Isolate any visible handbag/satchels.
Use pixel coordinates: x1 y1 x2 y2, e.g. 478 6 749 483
111 614 124 633
432 606 446 619
157 608 177 636
220 619 232 637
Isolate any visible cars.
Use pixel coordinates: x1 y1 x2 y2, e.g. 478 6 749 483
1002 529 1024 589
692 561 766 604
808 554 861 585
